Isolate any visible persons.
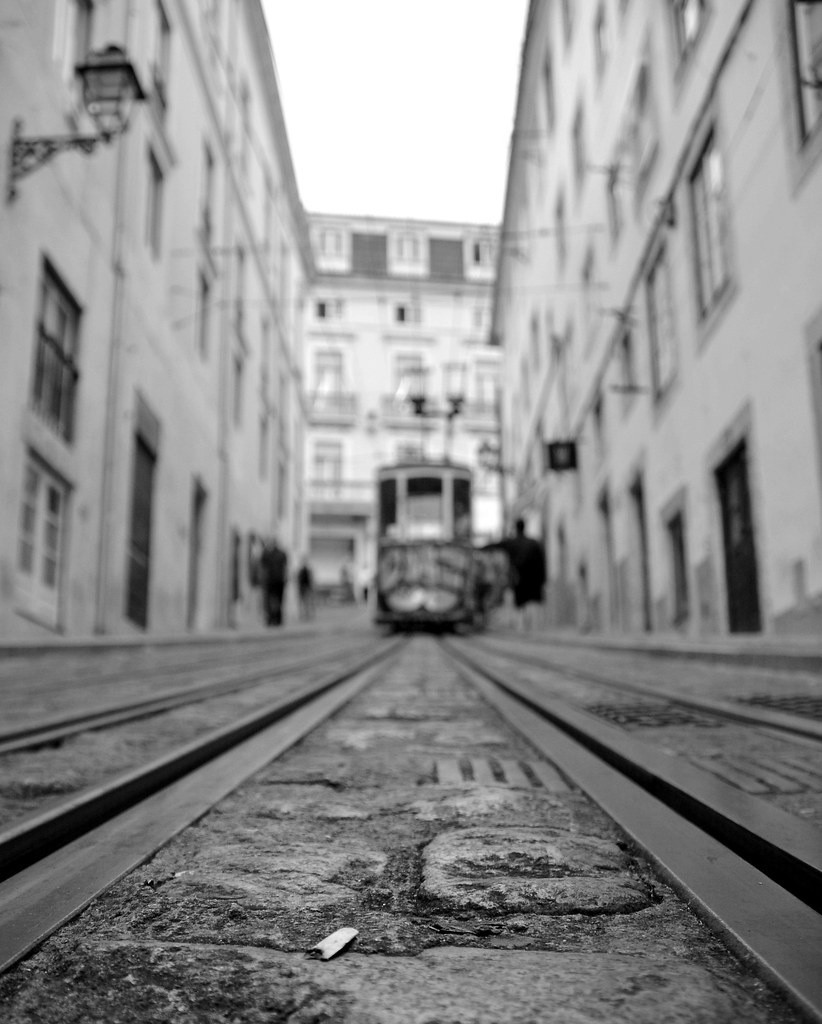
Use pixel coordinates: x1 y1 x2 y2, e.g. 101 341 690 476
262 538 372 626
482 518 547 630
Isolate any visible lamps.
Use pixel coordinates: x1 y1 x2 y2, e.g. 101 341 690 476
11 43 146 201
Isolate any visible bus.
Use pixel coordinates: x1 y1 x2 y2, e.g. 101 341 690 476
369 461 481 635
